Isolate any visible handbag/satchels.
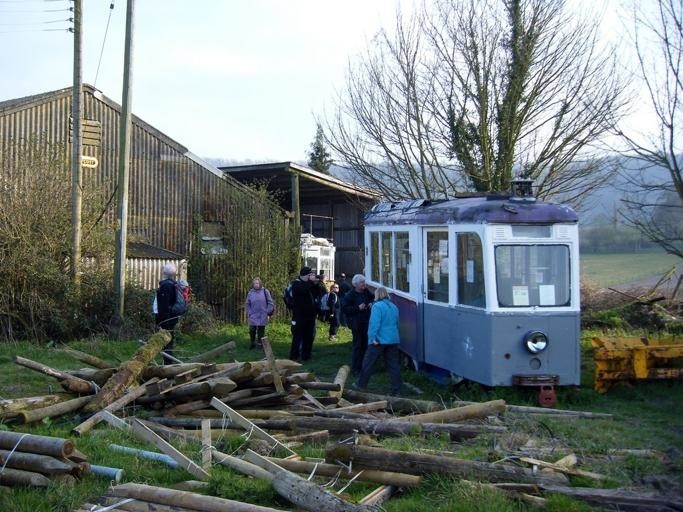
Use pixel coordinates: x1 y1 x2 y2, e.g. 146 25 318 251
267 308 274 315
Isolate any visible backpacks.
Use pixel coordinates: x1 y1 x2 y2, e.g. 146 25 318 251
321 293 337 310
283 282 294 307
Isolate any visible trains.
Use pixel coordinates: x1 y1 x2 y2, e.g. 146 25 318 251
360 176 585 397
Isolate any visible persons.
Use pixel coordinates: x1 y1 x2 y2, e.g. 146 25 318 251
154 263 179 365
290 267 375 380
350 286 403 398
244 276 274 351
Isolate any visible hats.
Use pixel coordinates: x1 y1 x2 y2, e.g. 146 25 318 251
300 267 312 276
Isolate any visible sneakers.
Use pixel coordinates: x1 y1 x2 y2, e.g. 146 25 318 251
352 383 368 393
328 338 338 342
